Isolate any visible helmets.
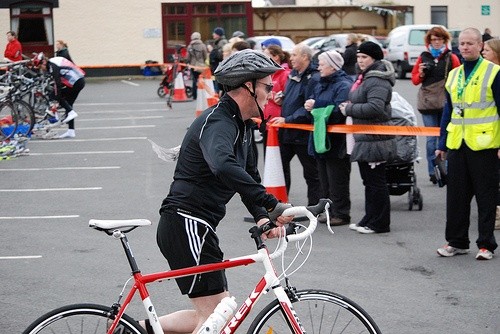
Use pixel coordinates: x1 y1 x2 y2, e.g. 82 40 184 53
34 52 44 67
213 49 284 87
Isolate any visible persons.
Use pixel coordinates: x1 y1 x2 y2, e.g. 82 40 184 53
55 40 72 61
203 26 293 162
336 40 392 235
5 31 23 66
341 33 366 76
303 51 352 225
480 27 500 68
106 49 295 334
267 43 321 206
435 27 500 260
412 26 460 185
34 53 85 137
185 32 208 99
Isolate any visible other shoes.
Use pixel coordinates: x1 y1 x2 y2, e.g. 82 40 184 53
318 214 331 222
62 110 78 122
60 129 75 138
329 217 350 226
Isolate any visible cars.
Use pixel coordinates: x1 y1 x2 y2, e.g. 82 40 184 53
246 33 387 62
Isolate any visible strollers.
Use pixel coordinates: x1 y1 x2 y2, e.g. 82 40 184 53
157 52 193 98
363 91 423 211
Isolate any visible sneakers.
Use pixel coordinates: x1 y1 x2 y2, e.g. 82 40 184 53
475 248 494 260
349 224 362 231
437 245 470 256
358 226 375 233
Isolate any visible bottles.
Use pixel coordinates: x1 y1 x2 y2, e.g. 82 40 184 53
197 296 238 334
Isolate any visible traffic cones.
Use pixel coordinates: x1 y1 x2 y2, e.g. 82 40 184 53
196 69 218 117
264 123 289 204
172 72 188 102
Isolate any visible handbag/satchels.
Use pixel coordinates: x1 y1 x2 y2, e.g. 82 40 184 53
417 79 446 114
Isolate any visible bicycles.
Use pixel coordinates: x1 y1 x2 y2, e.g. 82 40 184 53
0 52 63 144
21 199 382 334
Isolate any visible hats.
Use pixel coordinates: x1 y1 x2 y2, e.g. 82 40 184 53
357 41 384 60
322 51 344 70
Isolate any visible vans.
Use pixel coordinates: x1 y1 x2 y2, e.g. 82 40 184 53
384 23 452 79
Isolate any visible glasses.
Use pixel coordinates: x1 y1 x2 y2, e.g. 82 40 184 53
256 82 274 92
431 38 444 42
